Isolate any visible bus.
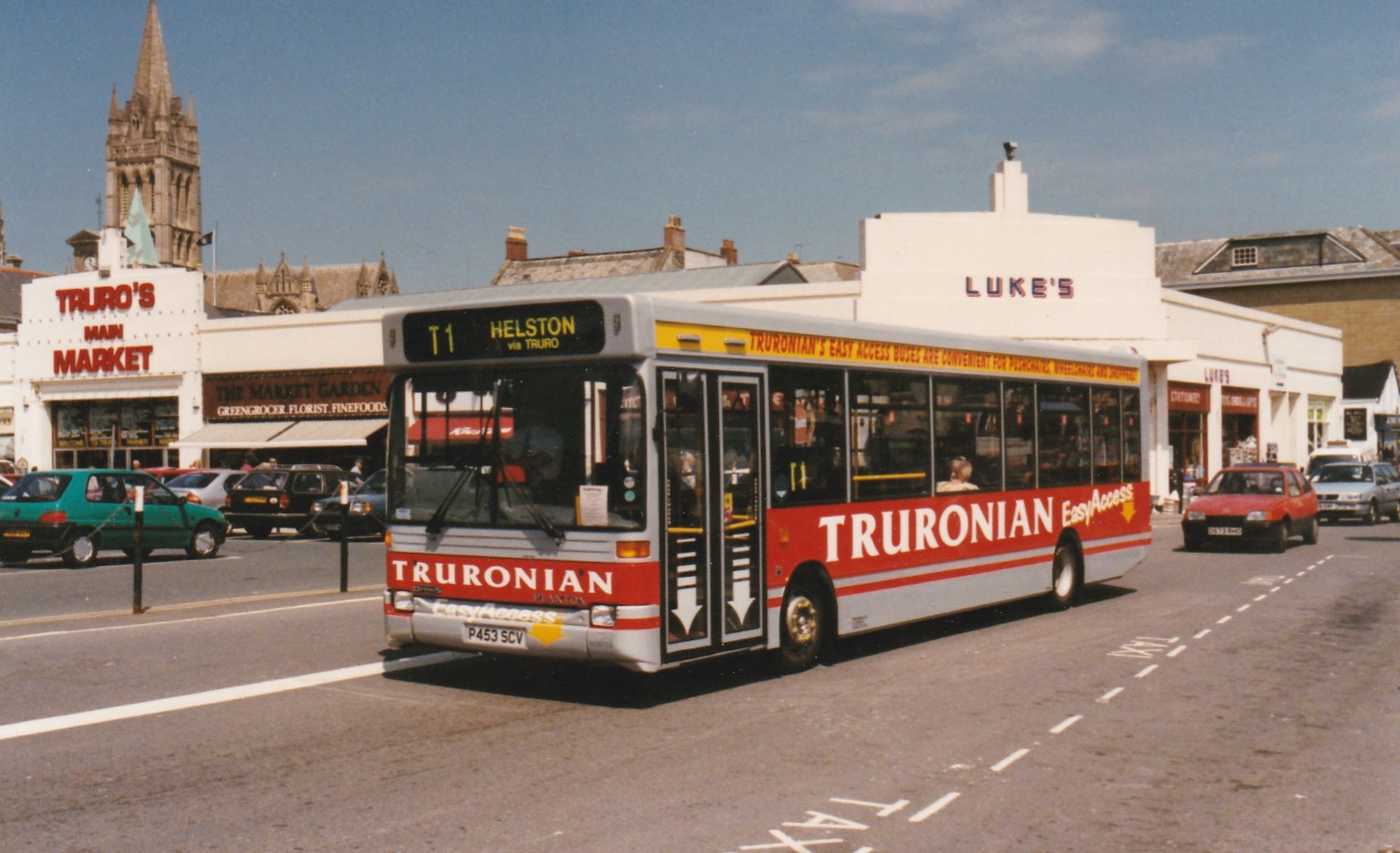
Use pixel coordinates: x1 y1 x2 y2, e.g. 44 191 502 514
382 295 1154 676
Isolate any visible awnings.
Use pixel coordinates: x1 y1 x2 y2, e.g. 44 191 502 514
167 417 389 449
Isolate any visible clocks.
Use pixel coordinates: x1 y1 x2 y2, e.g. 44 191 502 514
83 255 97 271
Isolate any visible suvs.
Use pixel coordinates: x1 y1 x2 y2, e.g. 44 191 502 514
223 464 363 539
1306 460 1400 525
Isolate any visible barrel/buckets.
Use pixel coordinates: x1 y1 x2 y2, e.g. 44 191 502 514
1163 498 1180 515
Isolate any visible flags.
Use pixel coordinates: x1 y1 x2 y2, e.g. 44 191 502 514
124 188 160 267
195 232 213 246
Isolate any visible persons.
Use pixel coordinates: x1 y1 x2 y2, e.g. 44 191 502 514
190 457 277 470
29 466 41 497
87 465 99 494
349 457 365 486
491 400 563 485
1227 472 1247 493
935 454 980 492
133 459 142 472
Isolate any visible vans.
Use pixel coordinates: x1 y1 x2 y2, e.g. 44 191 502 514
1300 446 1377 483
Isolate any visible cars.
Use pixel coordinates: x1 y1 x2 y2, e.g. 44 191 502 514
86 467 214 504
0 469 228 569
311 467 427 541
148 469 246 536
0 473 59 501
1180 464 1319 554
